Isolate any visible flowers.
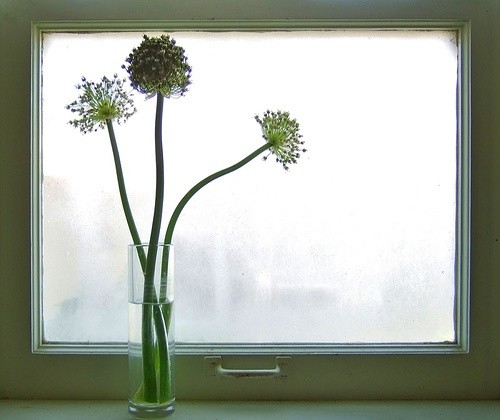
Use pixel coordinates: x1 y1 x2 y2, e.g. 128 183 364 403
63 32 306 402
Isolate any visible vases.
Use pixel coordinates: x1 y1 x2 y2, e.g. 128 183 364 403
126 244 175 418
